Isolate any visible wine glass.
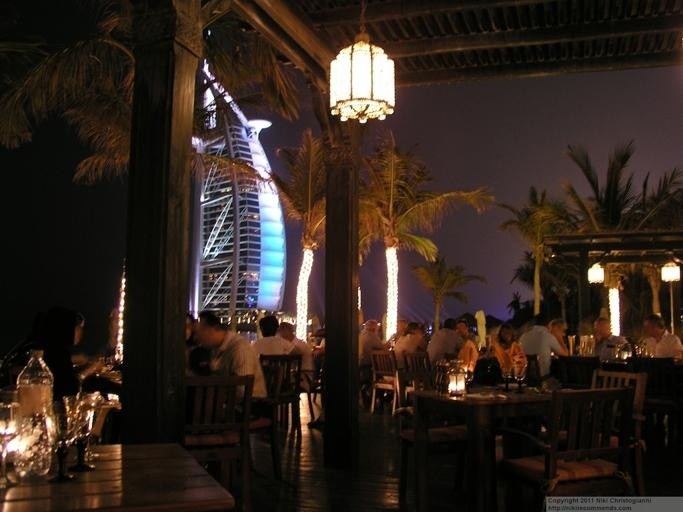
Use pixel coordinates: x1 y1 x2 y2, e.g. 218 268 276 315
510 363 527 393
50 391 102 481
500 365 512 392
0 400 19 488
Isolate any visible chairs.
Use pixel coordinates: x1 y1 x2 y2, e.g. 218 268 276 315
1 333 682 512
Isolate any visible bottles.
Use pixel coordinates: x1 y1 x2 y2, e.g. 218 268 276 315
15 349 54 474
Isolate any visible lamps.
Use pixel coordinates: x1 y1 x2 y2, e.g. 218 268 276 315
326 2 398 125
657 247 682 283
586 248 612 285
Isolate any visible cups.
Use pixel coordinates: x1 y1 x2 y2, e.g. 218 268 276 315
566 334 651 361
19 413 50 477
